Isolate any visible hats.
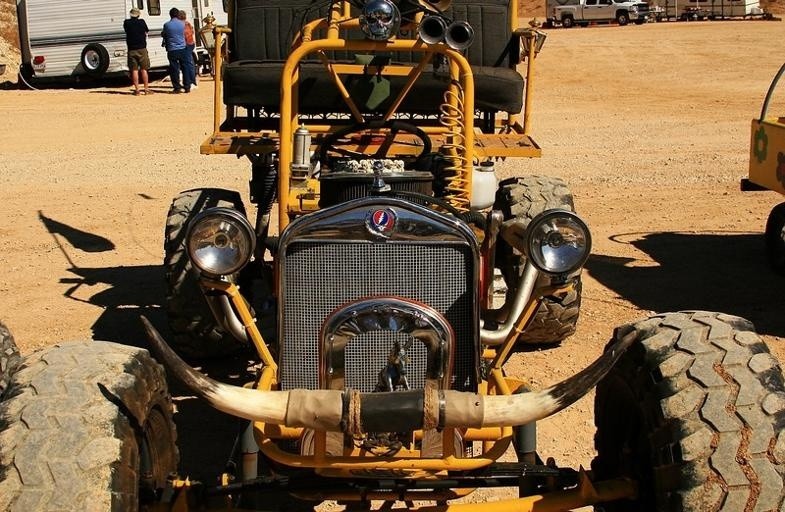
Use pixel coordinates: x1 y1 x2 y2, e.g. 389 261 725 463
130 8 141 17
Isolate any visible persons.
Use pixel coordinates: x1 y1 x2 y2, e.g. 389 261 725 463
178 9 198 89
123 6 154 96
160 6 191 93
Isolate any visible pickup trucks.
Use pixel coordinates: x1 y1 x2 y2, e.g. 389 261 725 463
553 0 649 28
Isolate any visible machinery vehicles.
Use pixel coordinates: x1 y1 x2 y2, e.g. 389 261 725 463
1 1 784 512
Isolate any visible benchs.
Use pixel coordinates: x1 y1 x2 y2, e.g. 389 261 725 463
223 0 524 132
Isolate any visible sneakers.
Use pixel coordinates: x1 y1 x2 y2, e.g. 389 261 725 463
168 89 190 93
190 82 198 89
134 89 154 96
